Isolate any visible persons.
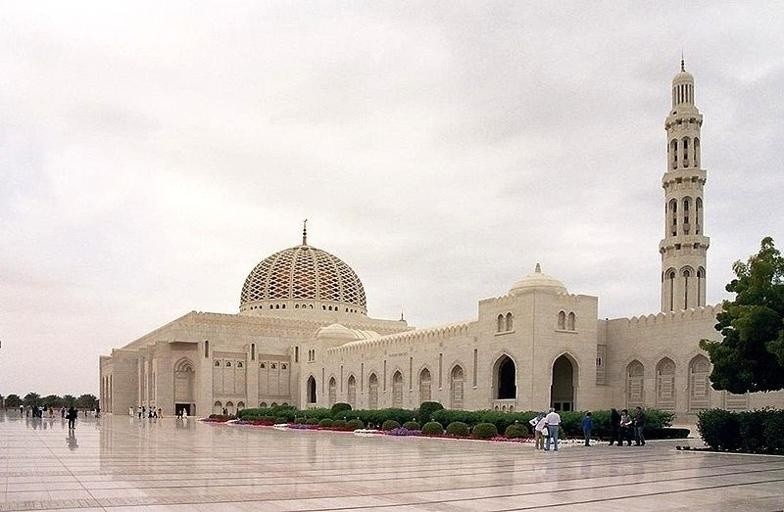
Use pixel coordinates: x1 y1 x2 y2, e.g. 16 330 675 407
183 408 187 418
19 404 101 429
178 408 183 418
222 407 225 415
129 406 163 419
530 411 547 450
582 412 593 447
225 408 228 415
609 407 646 446
544 408 562 451
